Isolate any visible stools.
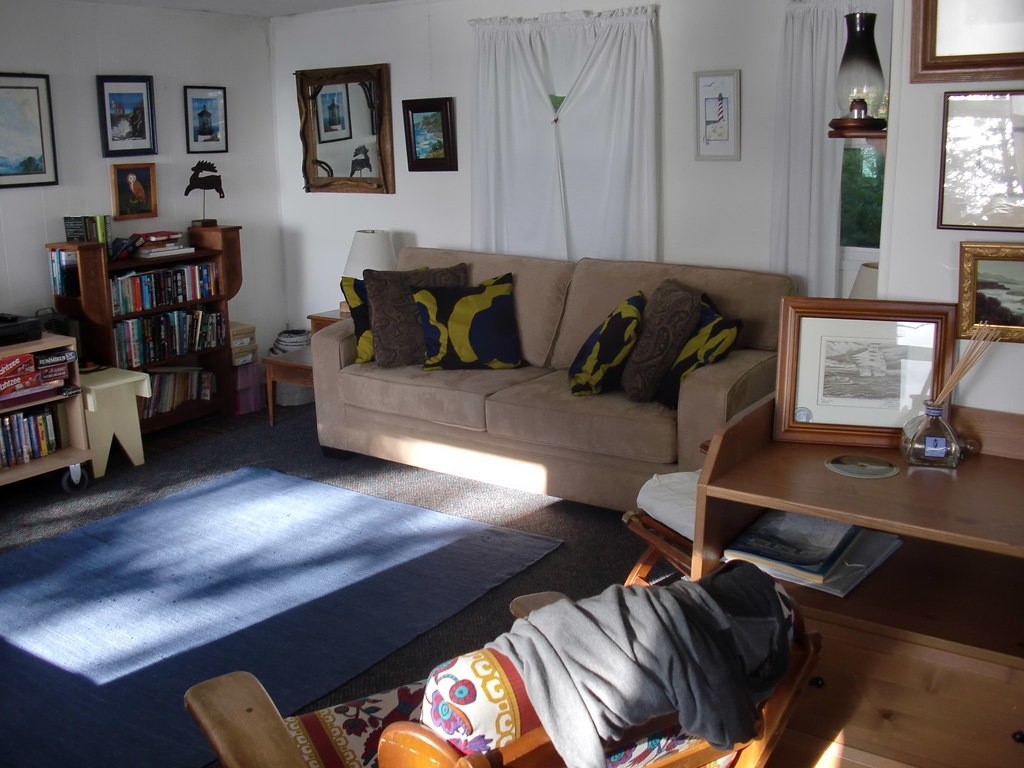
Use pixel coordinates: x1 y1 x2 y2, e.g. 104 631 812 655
56 366 152 480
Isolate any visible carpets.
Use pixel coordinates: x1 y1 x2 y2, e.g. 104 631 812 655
0 463 562 768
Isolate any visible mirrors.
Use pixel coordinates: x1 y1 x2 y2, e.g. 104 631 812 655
294 63 396 195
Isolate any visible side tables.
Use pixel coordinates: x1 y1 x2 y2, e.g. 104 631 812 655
261 308 352 429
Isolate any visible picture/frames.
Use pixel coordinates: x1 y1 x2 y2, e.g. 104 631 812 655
956 241 1024 343
772 296 957 447
110 162 159 222
692 69 741 161
0 72 59 190
183 85 229 154
908 0 1024 84
96 75 159 158
316 83 352 144
401 96 459 172
935 89 1024 232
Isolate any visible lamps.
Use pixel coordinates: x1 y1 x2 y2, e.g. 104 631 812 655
340 230 397 313
847 263 878 299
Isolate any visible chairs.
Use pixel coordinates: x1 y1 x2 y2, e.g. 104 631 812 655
185 592 819 768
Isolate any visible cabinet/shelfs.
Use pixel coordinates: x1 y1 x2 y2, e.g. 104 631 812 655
0 334 94 487
690 392 1024 768
44 226 243 434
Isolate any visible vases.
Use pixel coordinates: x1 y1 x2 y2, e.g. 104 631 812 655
836 13 886 117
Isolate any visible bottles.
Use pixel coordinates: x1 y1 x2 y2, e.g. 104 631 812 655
899 398 961 469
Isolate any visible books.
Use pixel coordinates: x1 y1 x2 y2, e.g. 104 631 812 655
0 215 259 473
722 498 904 599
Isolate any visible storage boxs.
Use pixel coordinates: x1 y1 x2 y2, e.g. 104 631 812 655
229 320 255 348
237 364 265 415
232 344 257 367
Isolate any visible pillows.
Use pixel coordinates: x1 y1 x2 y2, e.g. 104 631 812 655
362 263 467 368
620 278 699 401
340 266 429 365
661 293 739 404
567 290 643 398
411 272 529 370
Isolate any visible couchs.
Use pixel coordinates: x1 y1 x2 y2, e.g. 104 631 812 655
311 245 801 513
